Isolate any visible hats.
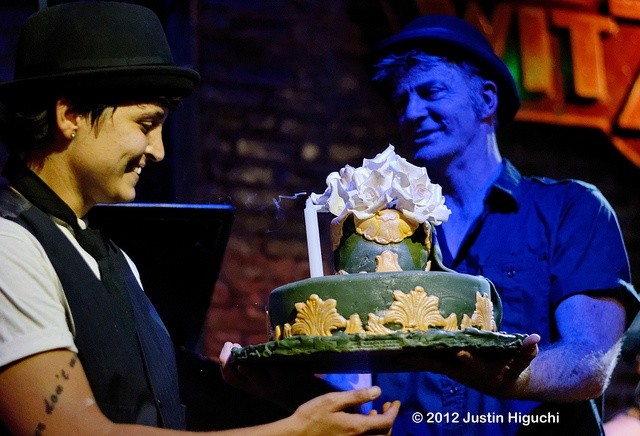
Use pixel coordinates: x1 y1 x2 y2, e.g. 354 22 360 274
341 16 520 118
1 1 200 86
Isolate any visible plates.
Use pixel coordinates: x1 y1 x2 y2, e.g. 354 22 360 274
232 330 528 373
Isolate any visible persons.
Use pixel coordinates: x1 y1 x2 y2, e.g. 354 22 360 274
324 15 640 435
0 0 402 436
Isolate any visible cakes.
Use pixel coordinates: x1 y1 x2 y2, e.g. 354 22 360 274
268 144 503 340
